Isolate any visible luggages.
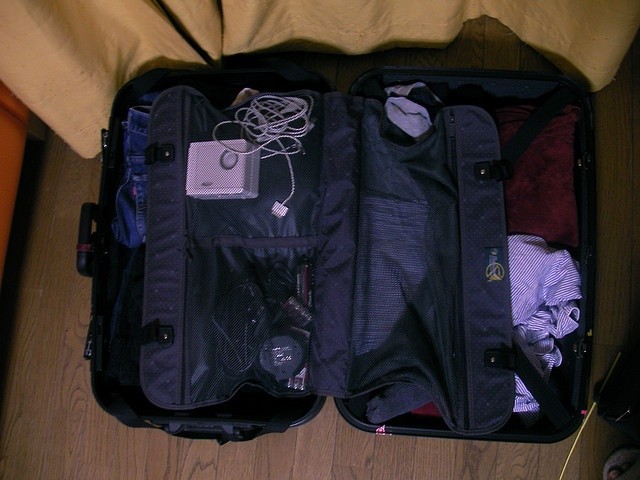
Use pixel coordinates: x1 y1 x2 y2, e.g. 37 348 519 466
68 12 604 446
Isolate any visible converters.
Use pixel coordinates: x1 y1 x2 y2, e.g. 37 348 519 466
258 333 304 384
183 137 262 201
